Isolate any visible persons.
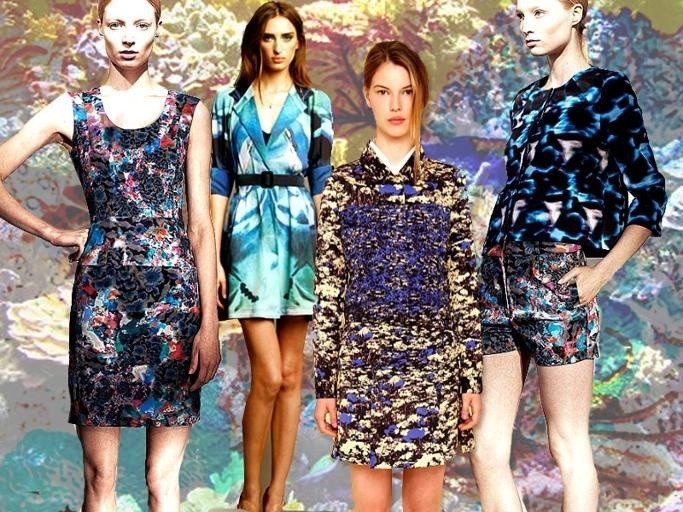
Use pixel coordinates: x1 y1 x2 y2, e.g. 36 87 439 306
213 0 334 511
0 1 223 511
312 36 484 512
465 0 666 511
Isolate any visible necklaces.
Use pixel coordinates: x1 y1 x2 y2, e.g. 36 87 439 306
261 79 287 114
